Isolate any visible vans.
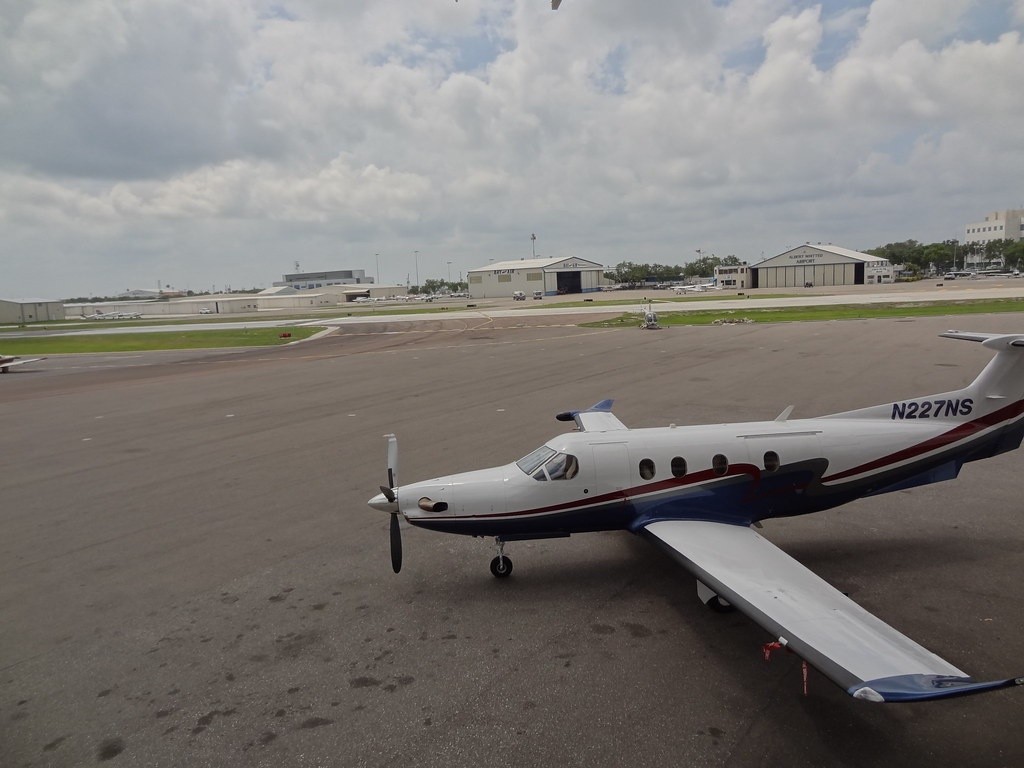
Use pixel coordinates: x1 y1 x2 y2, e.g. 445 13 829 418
532 290 543 299
512 291 526 301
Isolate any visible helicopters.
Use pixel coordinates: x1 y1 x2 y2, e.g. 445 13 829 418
638 296 671 330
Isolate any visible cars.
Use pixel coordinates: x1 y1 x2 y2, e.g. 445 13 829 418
944 272 955 280
199 307 212 314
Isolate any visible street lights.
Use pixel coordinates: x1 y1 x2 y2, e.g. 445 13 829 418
414 250 419 286
447 261 452 284
530 233 537 259
375 253 379 284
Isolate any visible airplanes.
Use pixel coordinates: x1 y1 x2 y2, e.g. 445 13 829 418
80 309 142 320
364 330 1023 706
